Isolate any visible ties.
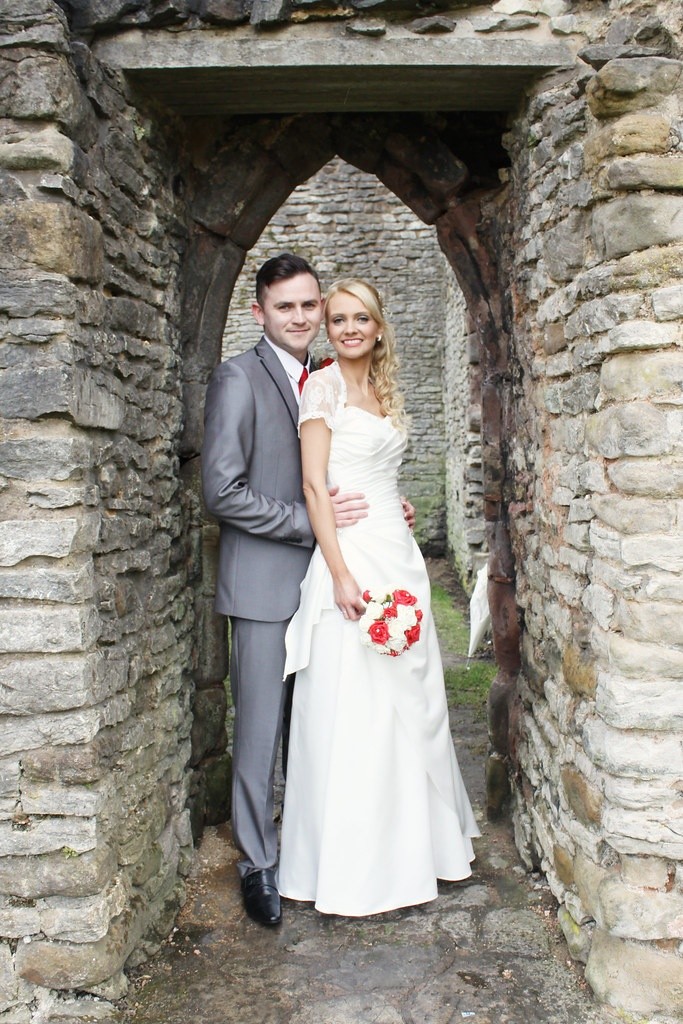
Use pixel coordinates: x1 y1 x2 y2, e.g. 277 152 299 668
298 367 309 396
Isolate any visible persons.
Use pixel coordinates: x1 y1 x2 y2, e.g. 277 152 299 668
201 253 417 926
272 278 483 917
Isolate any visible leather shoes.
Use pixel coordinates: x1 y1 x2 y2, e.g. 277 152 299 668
237 855 283 927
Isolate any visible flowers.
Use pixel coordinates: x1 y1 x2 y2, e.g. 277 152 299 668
358 590 423 657
